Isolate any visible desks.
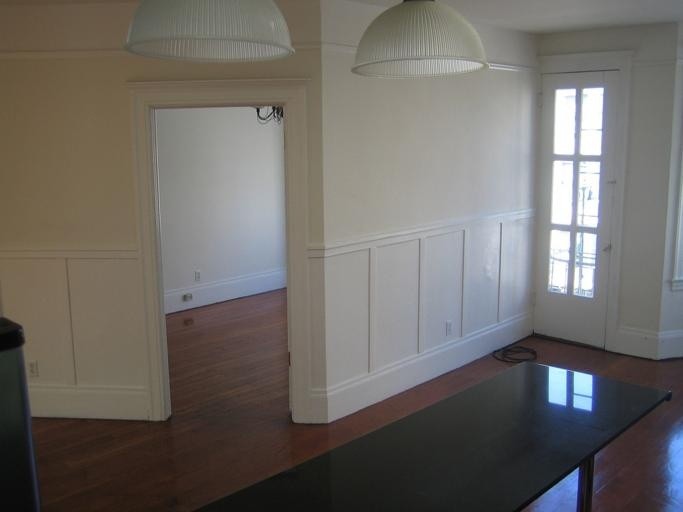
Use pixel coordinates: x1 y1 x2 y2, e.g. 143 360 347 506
195 362 662 511
1 316 39 511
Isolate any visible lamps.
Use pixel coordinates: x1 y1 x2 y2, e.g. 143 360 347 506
350 2 488 80
125 1 297 63
